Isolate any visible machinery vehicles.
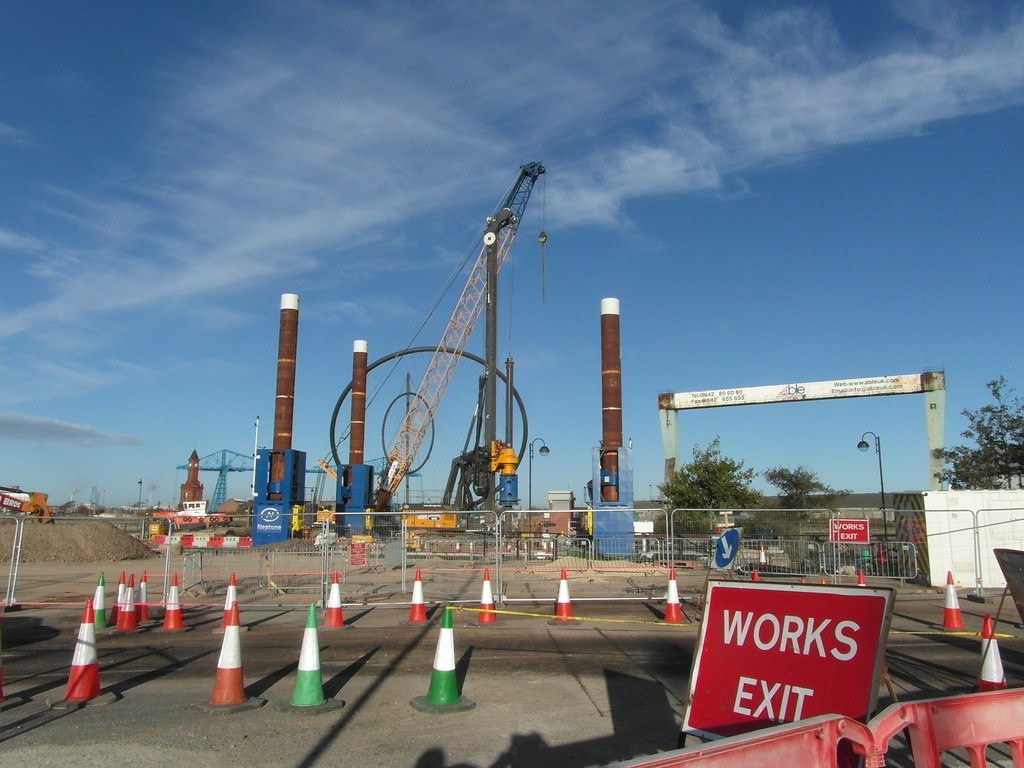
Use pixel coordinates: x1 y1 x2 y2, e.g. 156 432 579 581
311 162 547 539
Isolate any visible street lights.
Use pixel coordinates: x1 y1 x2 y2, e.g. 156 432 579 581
528 437 551 559
136 478 143 530
857 431 892 575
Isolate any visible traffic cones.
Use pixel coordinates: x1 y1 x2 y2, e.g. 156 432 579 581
821 578 827 585
408 601 476 713
190 600 268 713
44 598 121 711
942 570 968 632
857 569 868 587
759 544 767 566
152 574 193 634
90 572 116 630
0 608 26 712
753 569 761 581
317 572 356 631
398 570 435 625
271 603 345 714
466 567 507 626
210 570 252 633
108 569 163 635
655 568 691 625
800 577 807 583
976 613 1010 693
547 569 582 625
